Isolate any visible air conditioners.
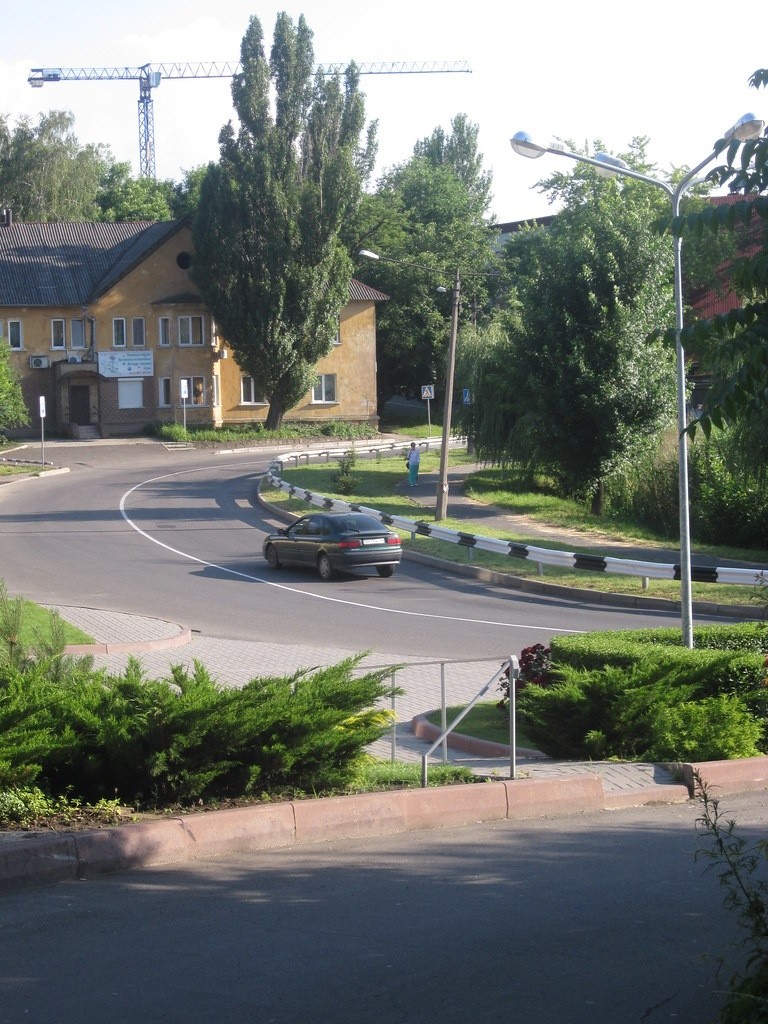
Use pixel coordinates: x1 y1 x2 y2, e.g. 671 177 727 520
32 358 48 368
68 355 81 362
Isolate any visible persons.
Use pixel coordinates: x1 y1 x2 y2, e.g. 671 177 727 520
406 442 420 486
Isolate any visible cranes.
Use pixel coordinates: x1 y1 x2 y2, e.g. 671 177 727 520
23 57 474 188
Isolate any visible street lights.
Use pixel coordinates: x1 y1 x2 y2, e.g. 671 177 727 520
508 111 768 648
358 247 462 521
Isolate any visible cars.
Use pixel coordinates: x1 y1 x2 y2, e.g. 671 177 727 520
262 511 403 581
